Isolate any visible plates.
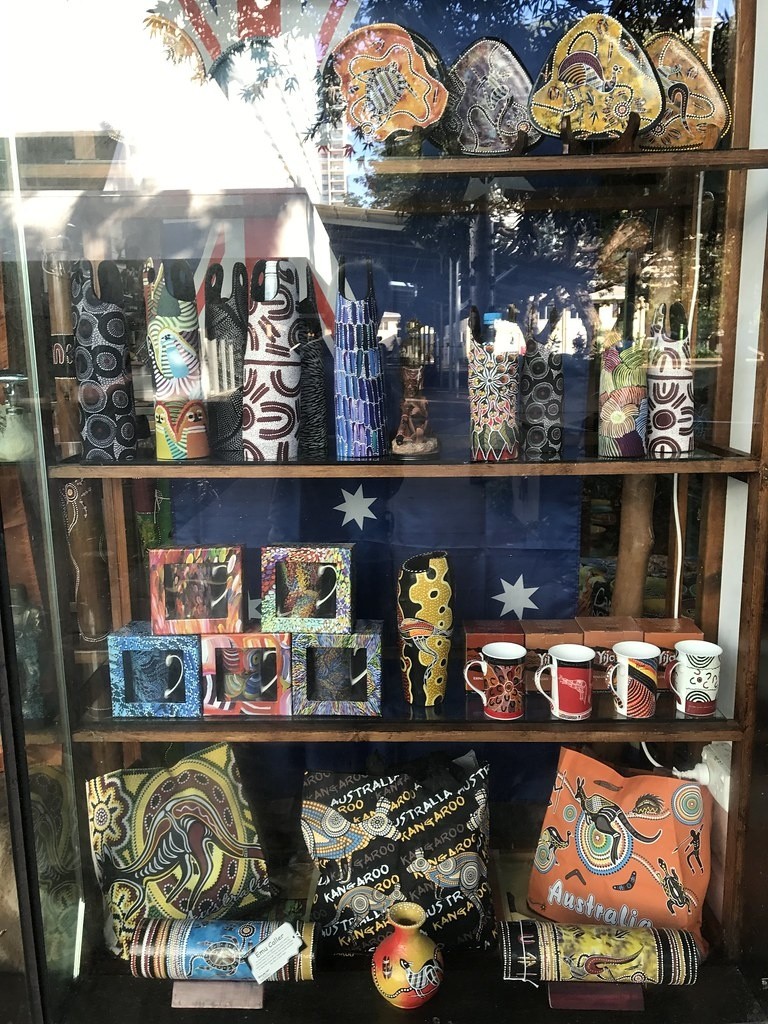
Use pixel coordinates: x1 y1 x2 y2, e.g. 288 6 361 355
326 13 733 157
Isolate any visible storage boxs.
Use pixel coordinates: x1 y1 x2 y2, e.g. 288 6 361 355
106 544 384 720
461 616 705 699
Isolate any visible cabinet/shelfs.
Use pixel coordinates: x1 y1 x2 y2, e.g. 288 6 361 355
0 0 768 1024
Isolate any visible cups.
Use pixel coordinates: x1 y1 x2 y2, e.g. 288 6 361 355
463 642 527 721
535 644 596 720
604 641 661 718
665 640 723 717
